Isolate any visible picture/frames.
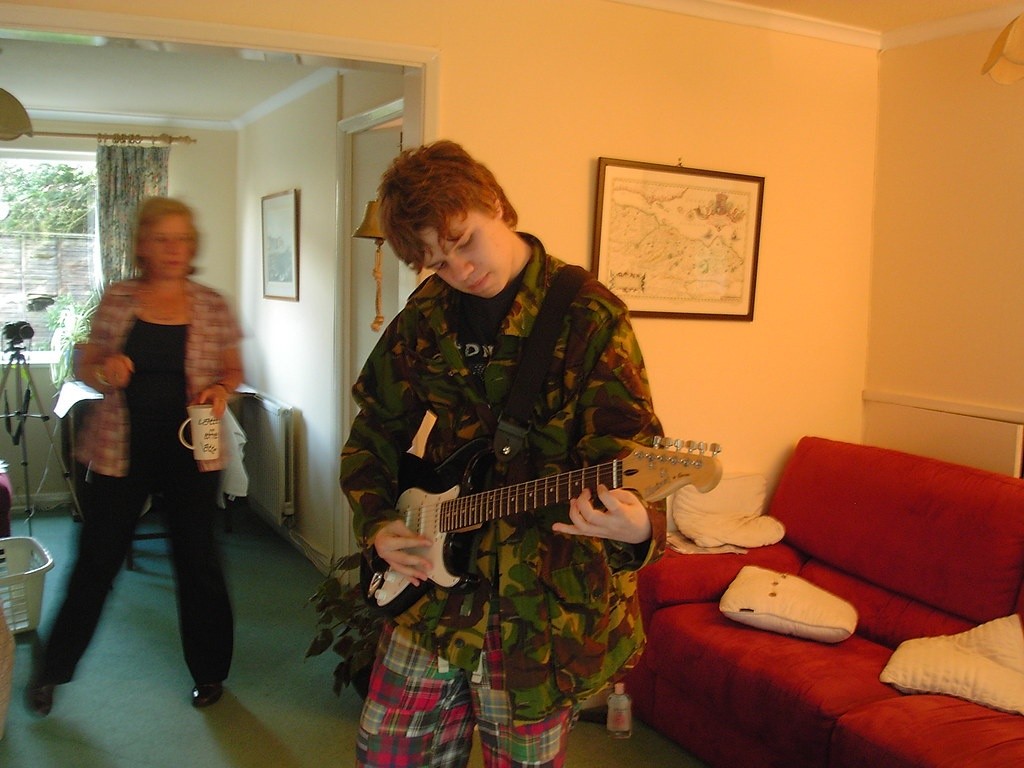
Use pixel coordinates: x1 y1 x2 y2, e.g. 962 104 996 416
260 188 299 302
591 156 765 321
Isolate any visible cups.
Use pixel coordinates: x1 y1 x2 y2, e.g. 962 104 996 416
180 404 221 460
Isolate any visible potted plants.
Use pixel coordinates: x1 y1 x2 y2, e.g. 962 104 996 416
304 538 400 699
43 289 100 392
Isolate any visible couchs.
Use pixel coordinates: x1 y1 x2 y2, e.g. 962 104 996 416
610 435 1024 768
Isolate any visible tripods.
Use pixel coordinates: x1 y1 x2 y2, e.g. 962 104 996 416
0 339 82 539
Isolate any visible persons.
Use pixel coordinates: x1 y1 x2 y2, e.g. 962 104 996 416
22 195 244 718
338 138 667 768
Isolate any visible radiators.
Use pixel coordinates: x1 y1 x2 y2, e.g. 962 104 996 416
241 395 294 527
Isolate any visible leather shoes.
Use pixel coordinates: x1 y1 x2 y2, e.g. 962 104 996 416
22 675 55 718
192 682 223 707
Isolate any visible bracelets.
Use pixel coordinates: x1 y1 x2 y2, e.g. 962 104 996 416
93 365 111 388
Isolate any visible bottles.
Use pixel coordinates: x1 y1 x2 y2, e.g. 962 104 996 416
607 683 632 739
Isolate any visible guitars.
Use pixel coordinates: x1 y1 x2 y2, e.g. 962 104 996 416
359 433 724 616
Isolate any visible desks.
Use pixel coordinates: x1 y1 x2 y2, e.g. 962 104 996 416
49 370 253 570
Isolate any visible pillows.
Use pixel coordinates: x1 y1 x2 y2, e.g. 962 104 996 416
719 565 856 642
878 613 1024 717
673 476 785 548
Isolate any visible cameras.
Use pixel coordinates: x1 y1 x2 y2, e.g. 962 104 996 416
6 320 35 340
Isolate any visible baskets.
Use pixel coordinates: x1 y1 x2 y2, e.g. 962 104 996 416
0 535 55 635
